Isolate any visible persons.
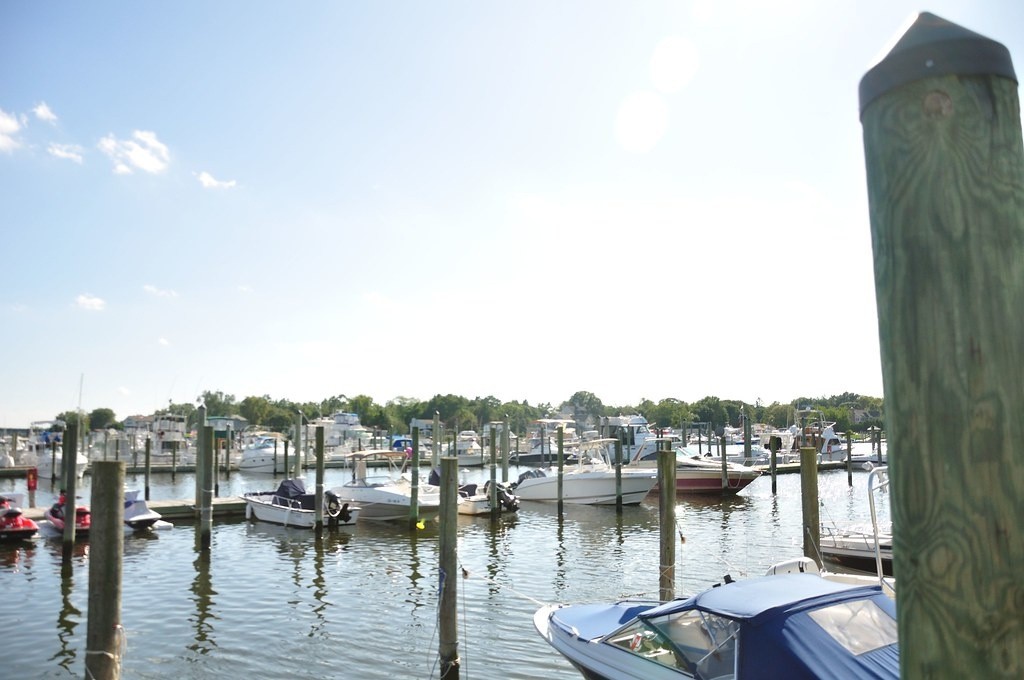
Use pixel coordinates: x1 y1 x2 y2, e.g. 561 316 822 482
58 489 67 518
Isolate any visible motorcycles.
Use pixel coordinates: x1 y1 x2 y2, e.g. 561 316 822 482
44 493 94 533
0 493 40 538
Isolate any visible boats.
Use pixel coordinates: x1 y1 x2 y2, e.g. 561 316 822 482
387 438 428 461
511 468 663 506
707 444 791 466
432 470 500 515
0 424 66 466
766 408 854 460
657 424 761 444
329 475 467 523
440 436 490 466
236 438 297 473
306 426 588 451
29 447 89 480
527 552 903 680
238 477 361 526
511 442 573 464
586 414 661 463
123 489 162 529
624 448 763 495
